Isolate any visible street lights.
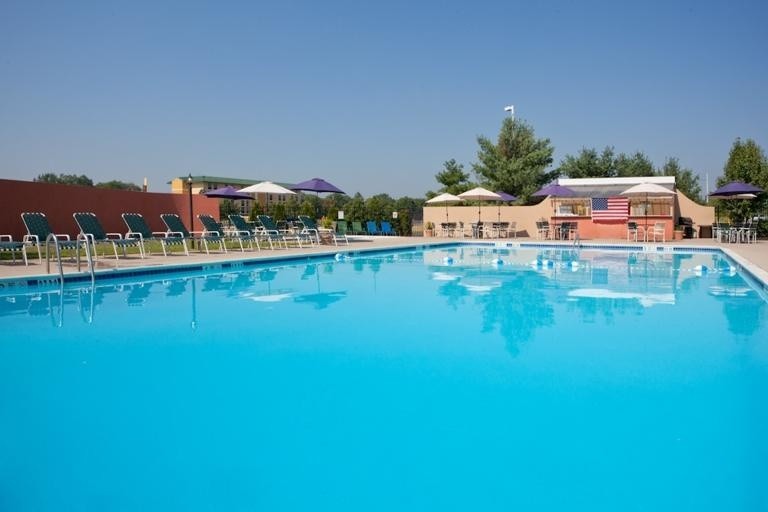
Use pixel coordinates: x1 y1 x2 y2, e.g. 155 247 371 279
185 172 195 249
190 278 197 333
503 104 515 139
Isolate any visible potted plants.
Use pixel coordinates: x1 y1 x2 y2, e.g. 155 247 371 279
673 225 685 240
422 221 435 237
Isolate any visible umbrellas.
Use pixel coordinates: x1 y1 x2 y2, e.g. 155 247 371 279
202 185 256 214
430 249 517 294
235 181 299 214
237 265 349 311
530 183 579 227
423 191 464 222
456 186 502 221
707 180 765 230
288 177 345 218
494 190 518 221
619 183 678 224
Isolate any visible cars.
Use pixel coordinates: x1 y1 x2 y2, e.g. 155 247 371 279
749 214 766 221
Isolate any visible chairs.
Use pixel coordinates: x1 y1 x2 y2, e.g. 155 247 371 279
712 216 758 244
197 214 349 254
1 212 204 267
625 219 665 243
348 219 400 236
535 216 579 241
438 220 518 239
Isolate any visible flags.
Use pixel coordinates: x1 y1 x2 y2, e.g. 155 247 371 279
591 196 629 223
